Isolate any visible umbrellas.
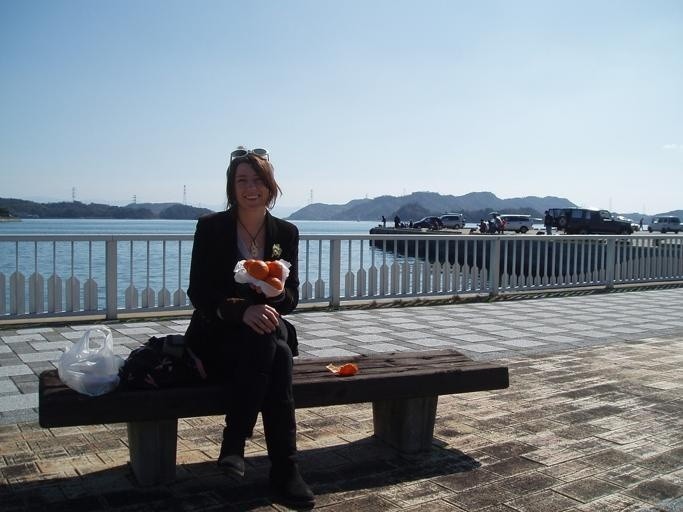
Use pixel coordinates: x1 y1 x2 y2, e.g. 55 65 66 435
487 212 500 216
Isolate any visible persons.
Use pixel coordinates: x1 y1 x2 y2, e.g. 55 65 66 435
478 215 505 235
638 217 644 231
381 216 386 223
393 216 400 228
183 146 318 508
543 210 553 235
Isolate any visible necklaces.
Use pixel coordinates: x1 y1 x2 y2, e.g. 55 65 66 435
235 217 266 257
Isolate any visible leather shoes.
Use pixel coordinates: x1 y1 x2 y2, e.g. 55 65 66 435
216 437 247 478
270 458 318 509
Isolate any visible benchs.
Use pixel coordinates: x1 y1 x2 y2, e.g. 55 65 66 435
39 349 509 487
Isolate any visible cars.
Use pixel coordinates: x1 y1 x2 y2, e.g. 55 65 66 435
412 216 445 229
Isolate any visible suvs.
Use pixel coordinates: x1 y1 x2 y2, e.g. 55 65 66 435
437 212 466 229
542 207 642 237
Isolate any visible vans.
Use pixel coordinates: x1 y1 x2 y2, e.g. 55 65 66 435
481 214 535 235
645 216 681 233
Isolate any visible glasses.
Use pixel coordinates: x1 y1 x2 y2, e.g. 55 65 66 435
229 148 270 164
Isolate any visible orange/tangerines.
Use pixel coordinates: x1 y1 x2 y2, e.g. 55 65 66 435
243 259 282 290
